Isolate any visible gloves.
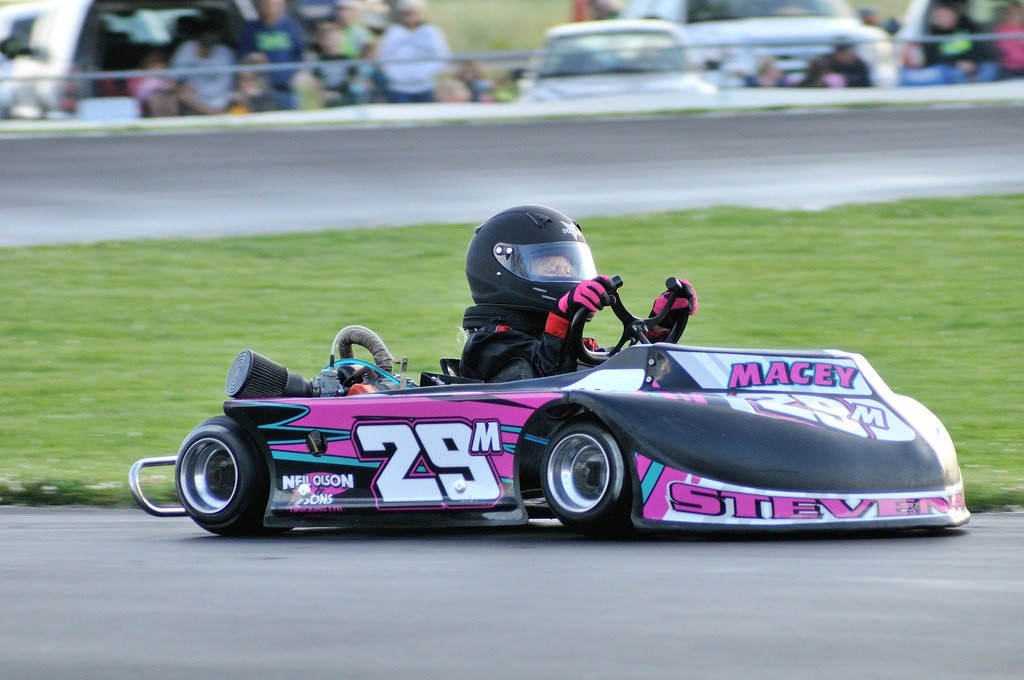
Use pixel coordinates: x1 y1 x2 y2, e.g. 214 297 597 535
648 280 699 335
554 275 616 320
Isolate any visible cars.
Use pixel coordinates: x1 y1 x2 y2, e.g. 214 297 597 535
618 0 897 87
508 18 721 101
892 0 1024 87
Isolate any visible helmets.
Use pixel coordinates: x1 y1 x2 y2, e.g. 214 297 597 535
461 204 598 339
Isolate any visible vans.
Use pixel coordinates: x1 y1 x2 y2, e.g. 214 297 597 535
0 0 248 114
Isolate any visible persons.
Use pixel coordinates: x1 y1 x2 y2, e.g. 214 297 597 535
739 1 1024 97
135 1 479 117
461 207 701 382
